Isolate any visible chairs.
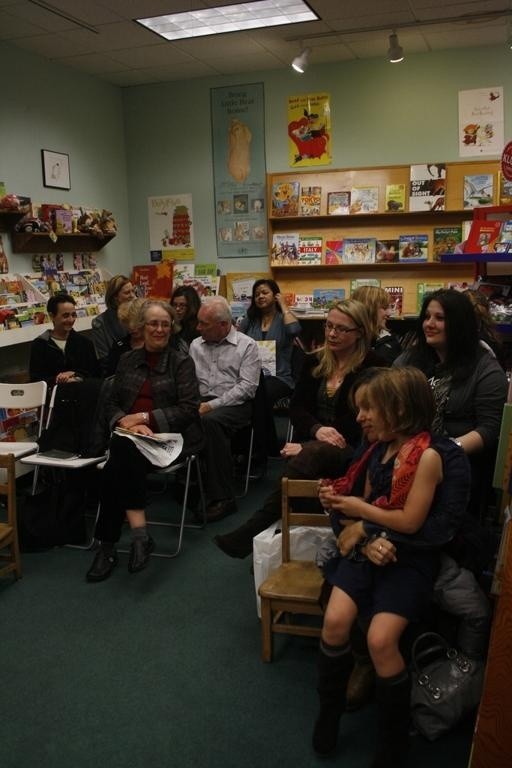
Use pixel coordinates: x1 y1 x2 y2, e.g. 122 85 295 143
0 303 512 557
256 474 346 661
2 453 23 583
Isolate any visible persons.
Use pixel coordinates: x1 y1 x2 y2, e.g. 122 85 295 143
318 364 473 705
23 276 509 598
312 364 470 768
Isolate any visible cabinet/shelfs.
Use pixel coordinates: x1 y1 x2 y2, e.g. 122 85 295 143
266 204 512 322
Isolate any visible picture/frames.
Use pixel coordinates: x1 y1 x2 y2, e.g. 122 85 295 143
41 147 72 189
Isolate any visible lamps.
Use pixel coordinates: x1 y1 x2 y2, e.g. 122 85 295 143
285 28 404 75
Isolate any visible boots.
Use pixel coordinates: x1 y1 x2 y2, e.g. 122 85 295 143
213 474 303 559
312 647 411 767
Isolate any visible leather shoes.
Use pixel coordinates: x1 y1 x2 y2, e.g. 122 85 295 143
87 548 119 580
128 533 155 573
203 498 237 520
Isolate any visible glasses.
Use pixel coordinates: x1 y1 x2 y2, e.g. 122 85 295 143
323 322 358 333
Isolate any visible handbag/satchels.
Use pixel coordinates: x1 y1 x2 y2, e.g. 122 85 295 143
252 519 336 618
409 632 485 740
18 483 86 552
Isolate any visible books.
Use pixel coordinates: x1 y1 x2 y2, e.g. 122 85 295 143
131 165 512 322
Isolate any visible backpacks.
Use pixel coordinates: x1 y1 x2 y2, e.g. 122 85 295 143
43 404 91 456
